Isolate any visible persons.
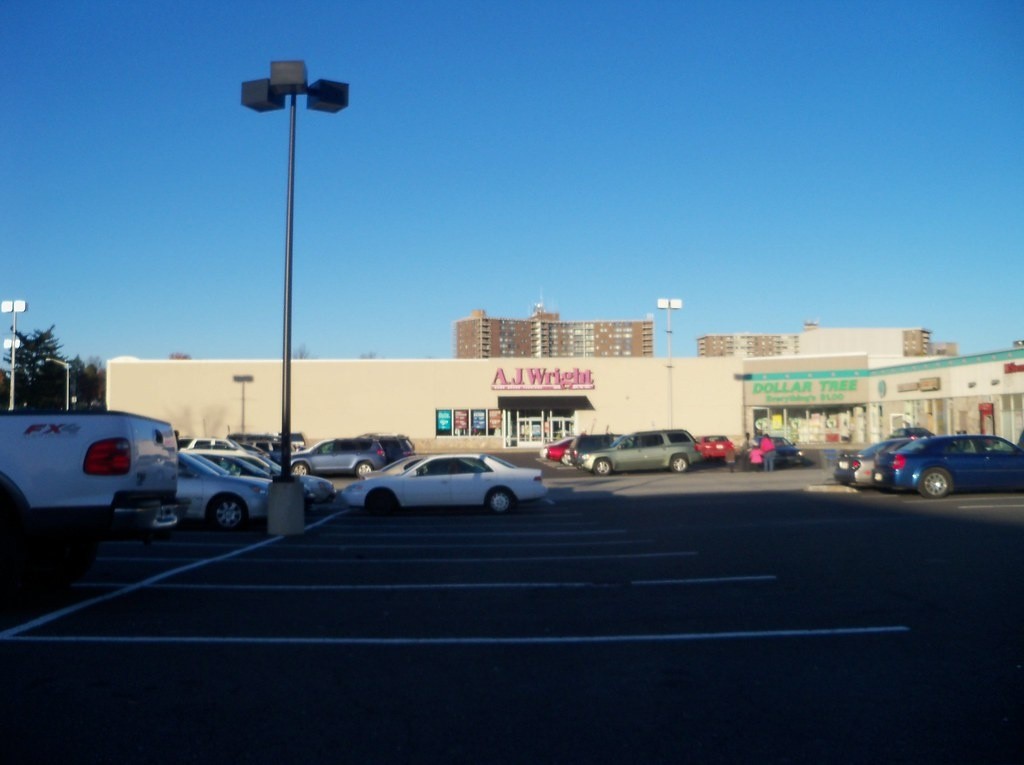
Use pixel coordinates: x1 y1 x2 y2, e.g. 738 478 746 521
727 447 737 473
749 446 764 473
741 433 758 470
760 433 776 473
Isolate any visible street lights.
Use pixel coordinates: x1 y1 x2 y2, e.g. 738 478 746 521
46 358 72 411
657 297 683 428
241 58 350 538
233 374 254 437
2 299 26 410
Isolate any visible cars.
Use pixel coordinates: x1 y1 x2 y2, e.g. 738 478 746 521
747 434 805 471
834 427 1024 499
695 434 736 467
361 453 488 475
165 431 337 533
340 452 550 517
539 429 707 478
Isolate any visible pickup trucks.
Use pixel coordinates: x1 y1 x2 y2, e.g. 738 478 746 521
0 410 182 601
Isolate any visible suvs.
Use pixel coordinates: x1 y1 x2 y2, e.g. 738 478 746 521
289 436 388 481
355 432 416 466
